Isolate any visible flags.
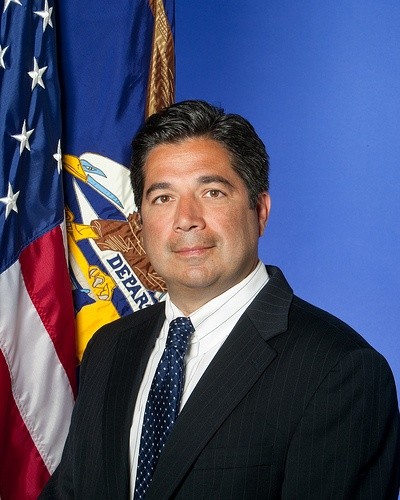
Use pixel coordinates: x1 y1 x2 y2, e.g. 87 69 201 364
0 0 176 500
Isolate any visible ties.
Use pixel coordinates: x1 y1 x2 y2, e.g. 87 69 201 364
132 316 195 500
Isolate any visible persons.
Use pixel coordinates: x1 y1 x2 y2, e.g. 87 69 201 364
37 98 400 500
